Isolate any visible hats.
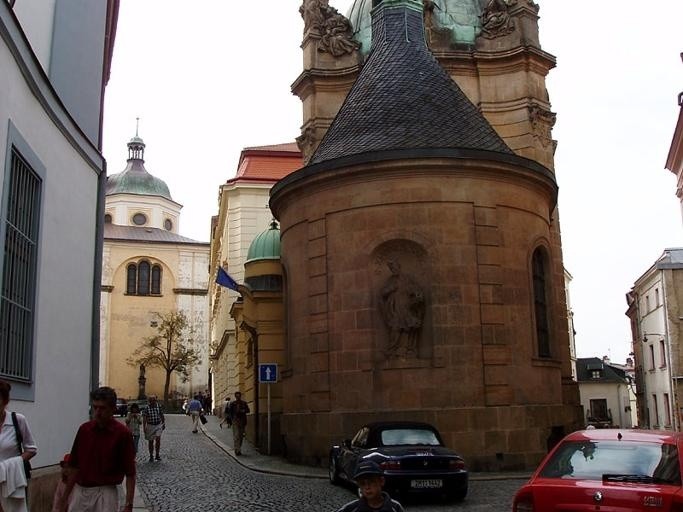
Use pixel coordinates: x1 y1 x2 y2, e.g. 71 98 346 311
354 461 384 477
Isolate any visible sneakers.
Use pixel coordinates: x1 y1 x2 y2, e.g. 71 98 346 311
150 455 162 461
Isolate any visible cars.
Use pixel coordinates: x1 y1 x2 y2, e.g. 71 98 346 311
512 427 683 512
328 421 470 505
115 398 129 418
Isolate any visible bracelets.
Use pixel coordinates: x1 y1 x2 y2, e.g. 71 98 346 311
125 503 134 507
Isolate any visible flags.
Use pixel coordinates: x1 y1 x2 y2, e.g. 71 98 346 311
215 268 237 290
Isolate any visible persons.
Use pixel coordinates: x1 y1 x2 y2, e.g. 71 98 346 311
337 461 405 512
0 380 38 512
379 260 421 350
55 386 136 512
186 390 230 433
480 0 516 40
230 392 250 456
125 403 142 463
318 7 362 57
142 395 165 462
